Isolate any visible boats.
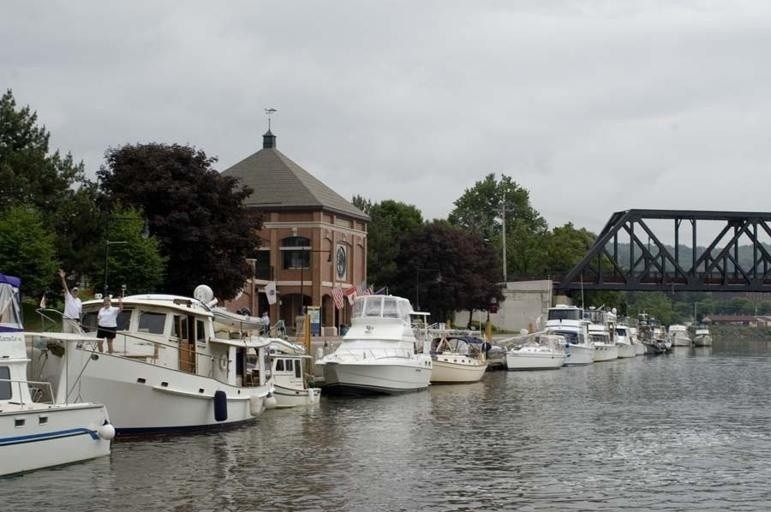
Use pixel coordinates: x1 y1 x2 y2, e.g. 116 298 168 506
423 335 492 383
247 319 321 409
24 284 277 437
1 273 116 477
314 293 433 396
503 303 691 371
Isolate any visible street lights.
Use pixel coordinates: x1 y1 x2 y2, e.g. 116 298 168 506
102 217 150 298
413 268 443 323
485 294 498 340
295 250 332 343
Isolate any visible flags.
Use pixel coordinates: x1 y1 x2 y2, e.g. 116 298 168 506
364 284 374 296
377 286 386 295
332 284 344 309
346 287 358 306
485 312 492 341
265 280 277 305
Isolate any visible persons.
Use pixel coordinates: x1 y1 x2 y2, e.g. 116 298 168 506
262 311 270 334
57 268 83 334
95 294 124 354
